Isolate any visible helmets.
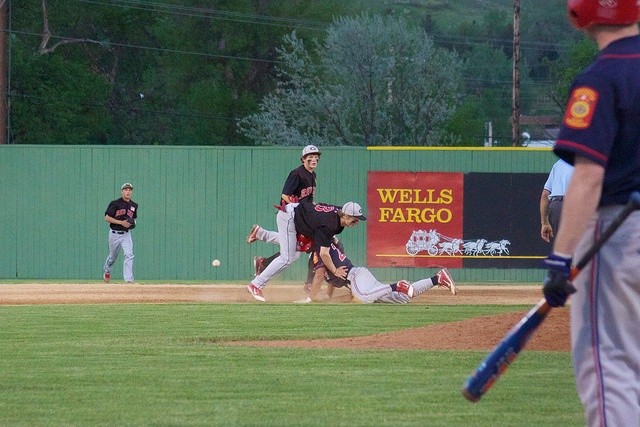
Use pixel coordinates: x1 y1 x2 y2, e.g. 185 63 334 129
297 233 315 251
566 0 640 30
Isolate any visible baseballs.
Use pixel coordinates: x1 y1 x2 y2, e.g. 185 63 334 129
212 259 220 267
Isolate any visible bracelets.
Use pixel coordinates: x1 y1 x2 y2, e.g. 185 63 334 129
333 268 338 275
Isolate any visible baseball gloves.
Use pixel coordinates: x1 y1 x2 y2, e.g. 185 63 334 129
122 218 136 229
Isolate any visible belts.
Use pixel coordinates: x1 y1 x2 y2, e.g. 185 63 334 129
550 195 564 201
112 229 129 234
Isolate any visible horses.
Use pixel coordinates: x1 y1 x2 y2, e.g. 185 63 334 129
439 240 464 257
485 242 510 256
485 239 511 256
462 239 481 256
467 239 487 256
438 239 462 257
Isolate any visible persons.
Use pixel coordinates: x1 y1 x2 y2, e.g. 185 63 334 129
102 181 138 284
539 157 576 243
246 196 367 304
293 232 456 304
254 145 322 292
542 1 640 427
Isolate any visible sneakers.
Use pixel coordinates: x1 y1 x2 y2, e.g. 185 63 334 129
303 281 324 294
247 223 260 243
396 280 414 298
253 255 267 278
435 268 455 294
104 271 110 282
246 281 266 301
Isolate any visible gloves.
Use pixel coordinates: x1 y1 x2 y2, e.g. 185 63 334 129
543 251 577 308
294 297 312 303
273 199 287 213
288 194 299 202
313 294 330 302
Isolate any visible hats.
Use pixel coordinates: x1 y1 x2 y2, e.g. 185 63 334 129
302 144 321 158
121 183 133 191
341 201 367 220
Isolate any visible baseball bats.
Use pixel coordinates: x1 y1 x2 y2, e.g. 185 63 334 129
460 191 640 402
